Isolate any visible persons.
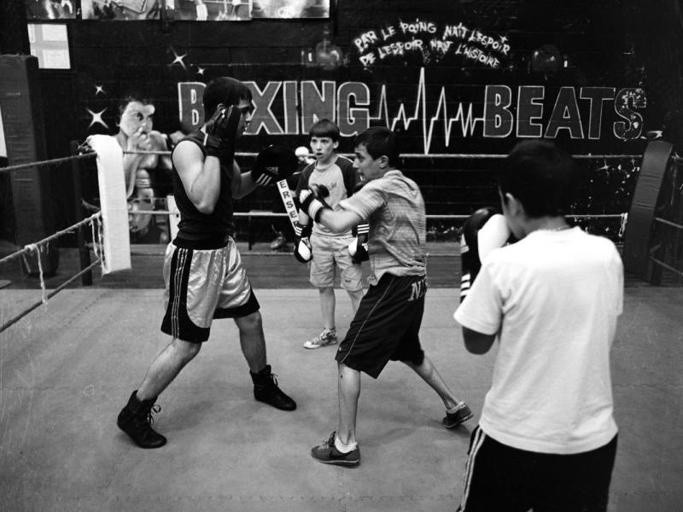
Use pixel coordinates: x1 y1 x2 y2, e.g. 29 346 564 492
104 92 185 242
452 141 626 511
292 143 315 168
299 127 474 468
296 119 365 351
116 78 298 449
252 131 298 250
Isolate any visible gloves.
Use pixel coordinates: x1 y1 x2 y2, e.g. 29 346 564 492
250 142 295 186
206 104 240 150
292 224 315 263
457 204 513 302
297 185 332 224
350 223 370 262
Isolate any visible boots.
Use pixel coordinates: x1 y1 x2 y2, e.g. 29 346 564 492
116 389 167 449
249 366 299 411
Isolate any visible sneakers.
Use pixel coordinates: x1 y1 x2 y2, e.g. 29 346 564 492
304 327 337 350
309 432 361 466
443 399 475 429
